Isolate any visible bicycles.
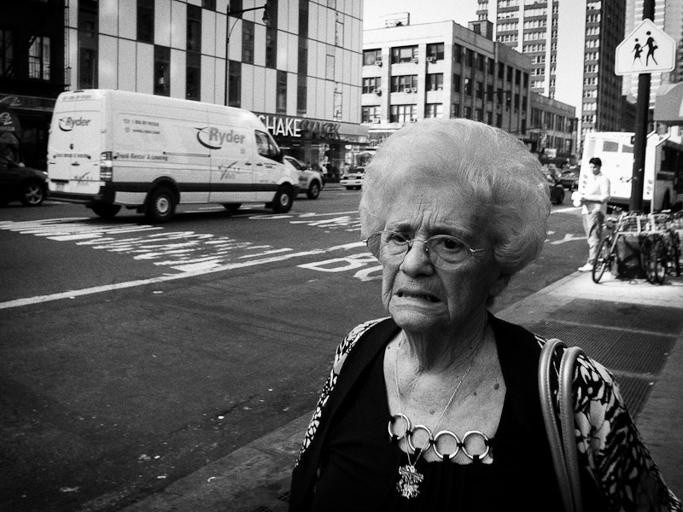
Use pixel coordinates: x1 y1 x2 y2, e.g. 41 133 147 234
592 199 683 286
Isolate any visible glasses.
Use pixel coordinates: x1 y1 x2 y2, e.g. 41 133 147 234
366 231 471 272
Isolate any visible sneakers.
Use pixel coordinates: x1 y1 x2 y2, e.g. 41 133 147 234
578 262 594 272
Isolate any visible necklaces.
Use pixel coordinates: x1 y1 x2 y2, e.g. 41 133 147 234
394 327 490 499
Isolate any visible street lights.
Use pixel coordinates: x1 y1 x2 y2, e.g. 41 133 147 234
220 2 275 106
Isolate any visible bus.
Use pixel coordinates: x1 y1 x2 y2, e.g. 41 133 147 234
336 139 381 187
578 130 683 214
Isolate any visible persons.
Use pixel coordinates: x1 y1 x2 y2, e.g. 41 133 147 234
290 118 682 508
570 156 612 273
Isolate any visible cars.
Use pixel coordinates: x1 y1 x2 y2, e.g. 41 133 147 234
285 152 325 202
0 151 48 206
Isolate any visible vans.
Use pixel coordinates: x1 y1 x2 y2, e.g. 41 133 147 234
43 86 302 222
